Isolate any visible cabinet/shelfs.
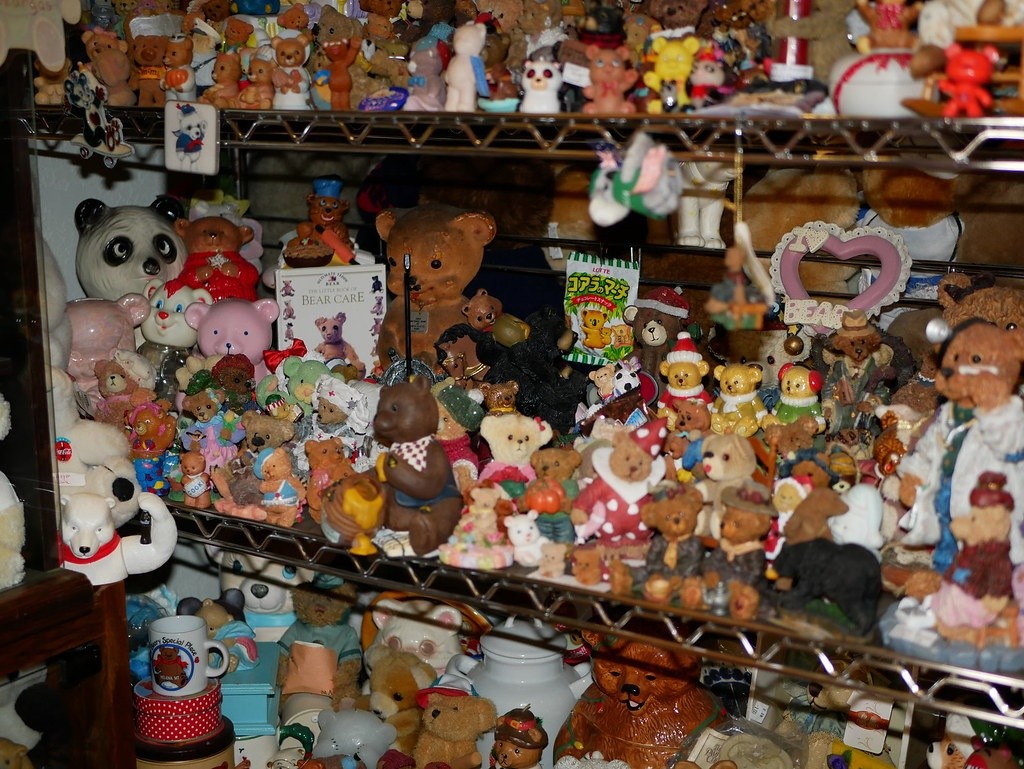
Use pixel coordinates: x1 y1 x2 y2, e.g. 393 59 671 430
0 46 1024 769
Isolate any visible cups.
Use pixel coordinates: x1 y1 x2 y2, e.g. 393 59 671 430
148 614 229 696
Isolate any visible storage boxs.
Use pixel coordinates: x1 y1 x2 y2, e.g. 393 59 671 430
133 676 223 742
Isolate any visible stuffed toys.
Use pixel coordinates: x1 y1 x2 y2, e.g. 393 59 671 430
0 395 28 588
412 673 497 769
228 410 294 474
353 643 437 755
276 579 362 712
480 412 553 488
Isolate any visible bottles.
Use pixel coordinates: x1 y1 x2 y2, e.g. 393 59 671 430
443 612 594 769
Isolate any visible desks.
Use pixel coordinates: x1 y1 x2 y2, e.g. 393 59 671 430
0 567 137 769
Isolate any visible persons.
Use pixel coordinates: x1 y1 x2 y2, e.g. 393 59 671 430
51 357 171 530
